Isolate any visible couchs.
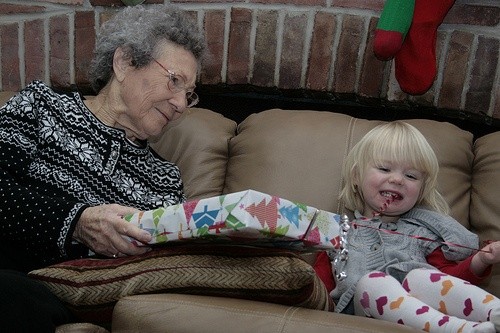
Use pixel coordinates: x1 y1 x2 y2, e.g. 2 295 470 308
1 90 500 332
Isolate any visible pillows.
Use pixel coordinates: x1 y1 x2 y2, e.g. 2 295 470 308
27 241 337 330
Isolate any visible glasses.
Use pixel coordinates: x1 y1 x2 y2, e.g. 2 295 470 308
149 52 200 108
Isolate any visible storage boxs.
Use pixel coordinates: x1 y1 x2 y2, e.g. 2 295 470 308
89 189 341 256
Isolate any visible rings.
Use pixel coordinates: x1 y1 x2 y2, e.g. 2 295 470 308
113 253 118 258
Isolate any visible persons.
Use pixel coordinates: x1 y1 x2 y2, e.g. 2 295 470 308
328 121 500 333
0 4 209 333
